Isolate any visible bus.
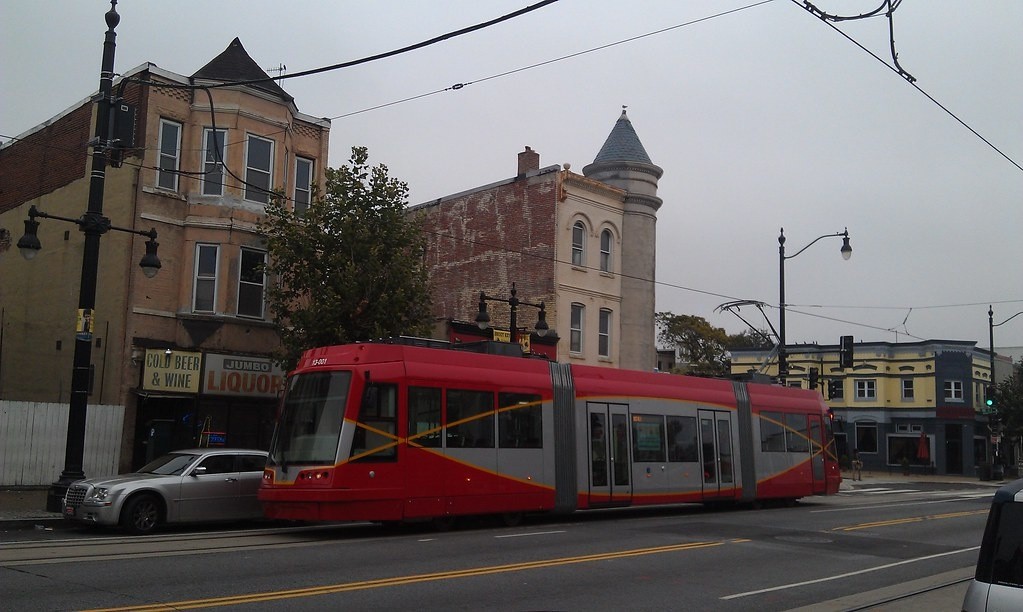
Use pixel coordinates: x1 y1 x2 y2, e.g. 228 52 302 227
266 343 843 525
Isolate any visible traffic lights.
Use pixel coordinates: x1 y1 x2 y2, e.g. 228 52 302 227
840 336 853 368
810 366 818 390
986 387 994 407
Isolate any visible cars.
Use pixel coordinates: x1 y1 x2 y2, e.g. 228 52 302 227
62 448 269 535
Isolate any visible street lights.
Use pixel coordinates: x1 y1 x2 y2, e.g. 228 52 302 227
475 281 549 345
17 204 163 512
778 226 852 387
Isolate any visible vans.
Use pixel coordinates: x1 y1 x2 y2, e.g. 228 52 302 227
961 477 1023 612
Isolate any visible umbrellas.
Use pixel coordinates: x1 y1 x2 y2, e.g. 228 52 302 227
917 432 928 472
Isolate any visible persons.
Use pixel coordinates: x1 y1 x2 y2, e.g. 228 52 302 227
83 309 91 332
